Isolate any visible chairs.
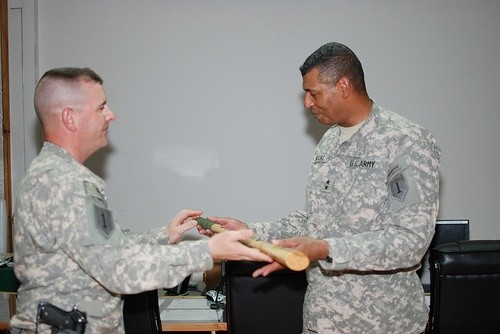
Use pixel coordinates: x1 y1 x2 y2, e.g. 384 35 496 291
425 239 500 334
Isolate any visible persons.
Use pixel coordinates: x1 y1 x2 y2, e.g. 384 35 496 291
196 41 441 334
9 67 273 334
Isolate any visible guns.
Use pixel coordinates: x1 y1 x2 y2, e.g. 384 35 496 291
36 300 88 334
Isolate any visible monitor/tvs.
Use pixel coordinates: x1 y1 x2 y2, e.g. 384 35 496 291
421 220 469 272
